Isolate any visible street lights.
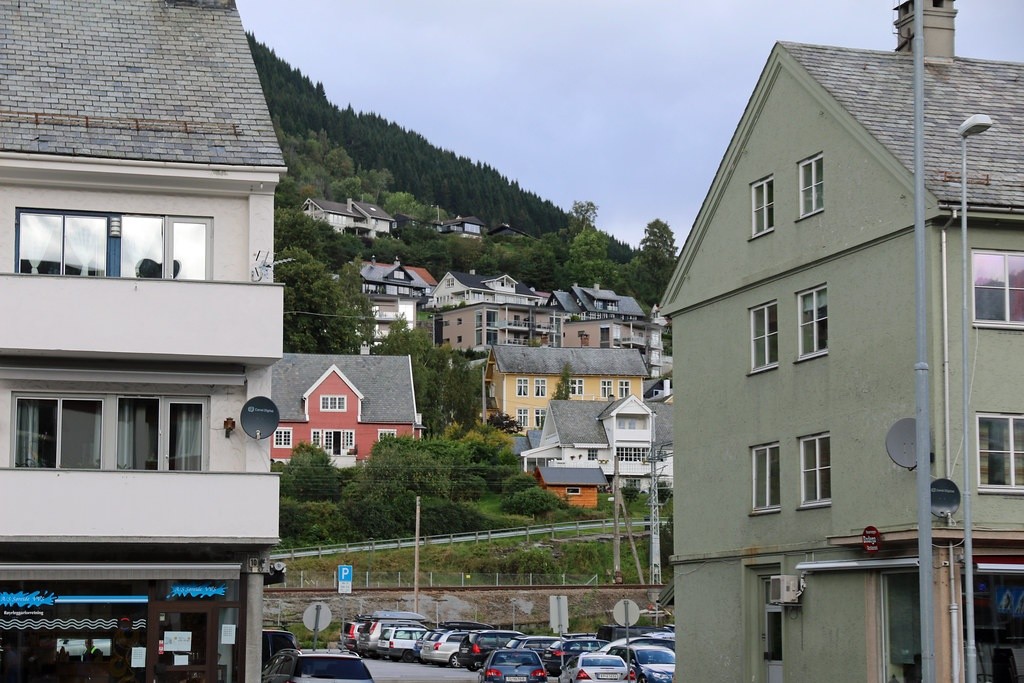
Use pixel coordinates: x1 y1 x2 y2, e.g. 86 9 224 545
956 113 991 683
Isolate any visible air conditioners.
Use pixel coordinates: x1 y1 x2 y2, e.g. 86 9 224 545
770 574 799 605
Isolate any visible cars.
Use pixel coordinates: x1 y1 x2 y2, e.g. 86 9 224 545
262 648 375 683
475 648 548 683
558 655 636 682
262 625 300 670
339 609 675 675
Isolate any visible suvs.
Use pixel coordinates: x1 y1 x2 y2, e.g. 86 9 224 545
607 646 675 683
